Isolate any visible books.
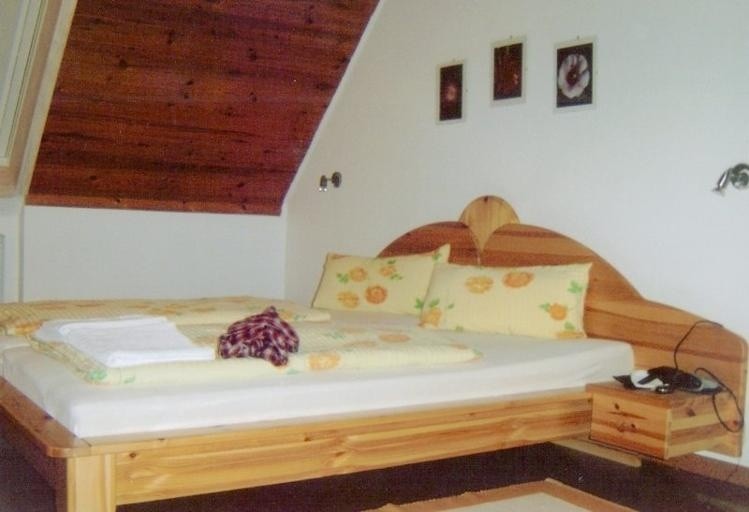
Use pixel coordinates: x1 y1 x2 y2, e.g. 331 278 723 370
586 375 735 460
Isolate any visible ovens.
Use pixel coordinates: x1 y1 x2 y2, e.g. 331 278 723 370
0 194 743 512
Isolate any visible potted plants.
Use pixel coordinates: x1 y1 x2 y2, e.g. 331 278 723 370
551 34 599 113
436 59 466 124
489 34 525 109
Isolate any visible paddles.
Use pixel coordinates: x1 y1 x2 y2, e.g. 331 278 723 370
358 478 637 512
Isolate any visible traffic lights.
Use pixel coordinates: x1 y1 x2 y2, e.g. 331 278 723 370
310 243 591 341
